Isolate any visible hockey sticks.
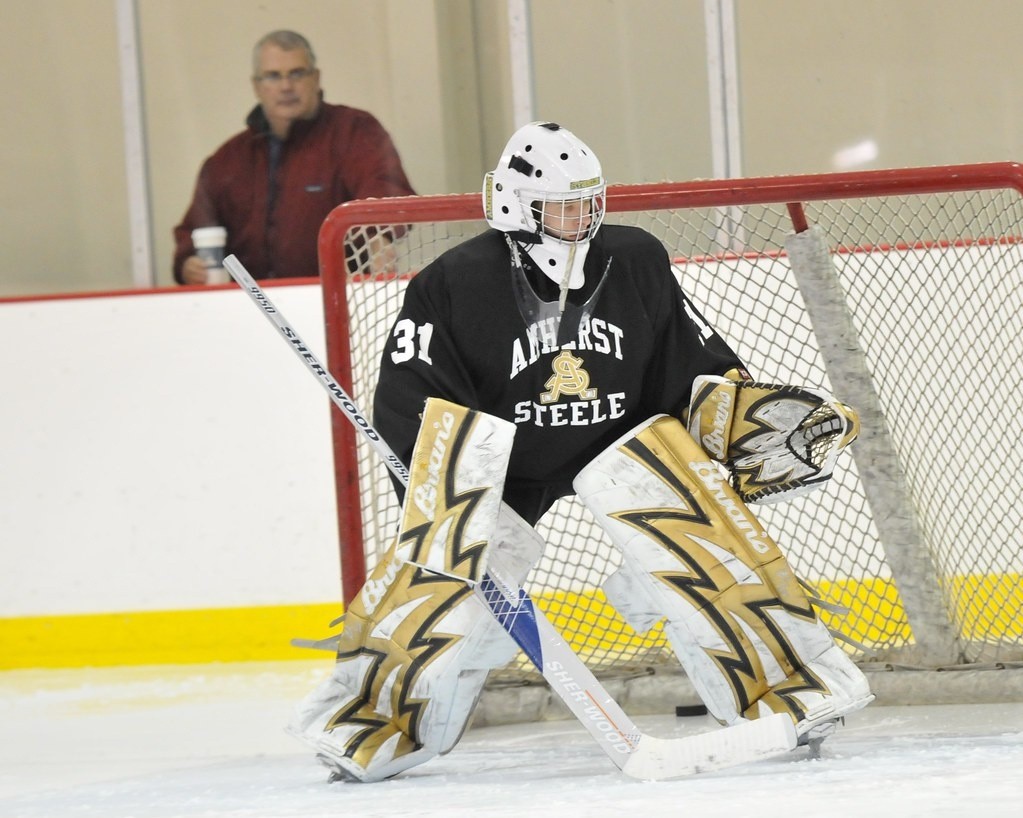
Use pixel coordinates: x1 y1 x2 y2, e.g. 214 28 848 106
219 251 800 782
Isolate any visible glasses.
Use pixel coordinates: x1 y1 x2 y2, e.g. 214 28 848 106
259 68 312 83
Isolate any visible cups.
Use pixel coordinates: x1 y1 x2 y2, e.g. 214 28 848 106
190 226 232 286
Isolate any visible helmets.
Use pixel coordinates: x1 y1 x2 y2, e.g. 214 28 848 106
481 120 607 290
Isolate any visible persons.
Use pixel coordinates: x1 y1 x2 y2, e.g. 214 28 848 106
172 30 417 286
286 121 876 783
